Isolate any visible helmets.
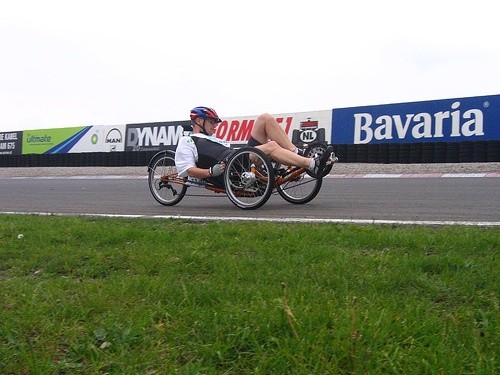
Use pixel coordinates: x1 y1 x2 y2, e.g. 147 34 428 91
190 106 222 123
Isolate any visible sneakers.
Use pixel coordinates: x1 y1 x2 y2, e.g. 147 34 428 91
297 148 306 155
313 146 333 175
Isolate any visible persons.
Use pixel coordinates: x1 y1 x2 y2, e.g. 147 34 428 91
175 106 333 192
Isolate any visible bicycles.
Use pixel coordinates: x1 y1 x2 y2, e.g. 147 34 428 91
148 141 339 210
